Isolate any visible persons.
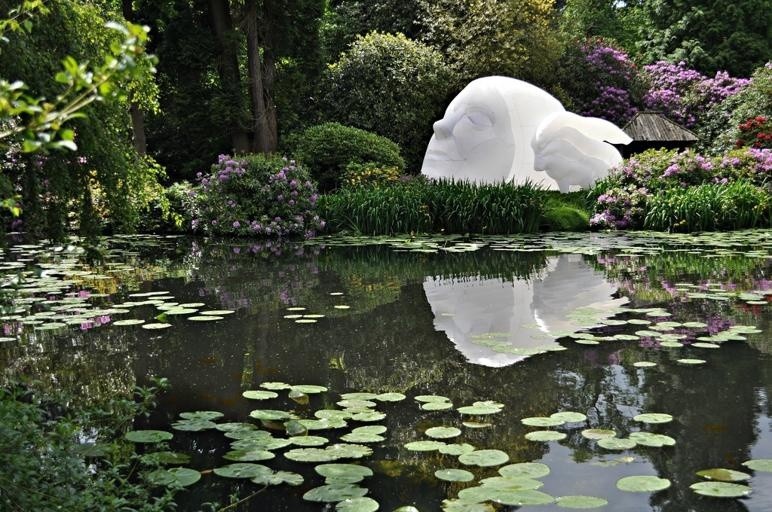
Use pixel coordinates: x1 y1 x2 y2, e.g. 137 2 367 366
421 272 557 371
417 75 573 190
